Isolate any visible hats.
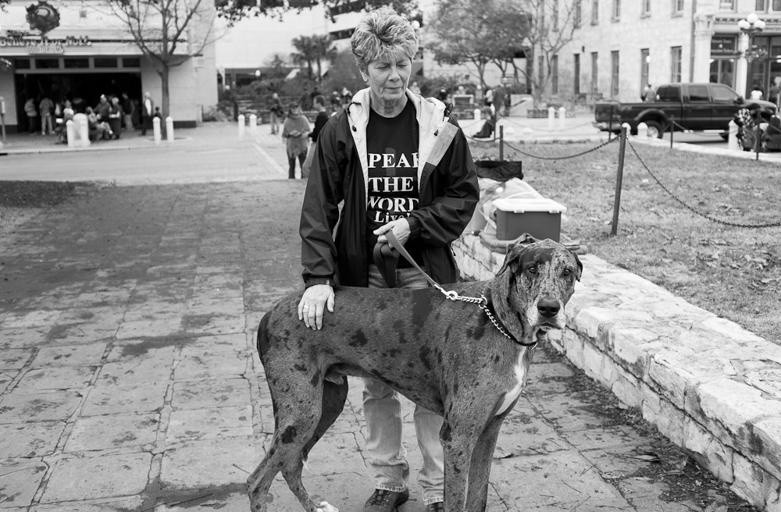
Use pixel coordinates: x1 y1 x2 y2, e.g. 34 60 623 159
290 102 299 109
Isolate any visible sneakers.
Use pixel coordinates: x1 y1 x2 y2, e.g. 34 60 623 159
427 501 445 512
364 487 410 512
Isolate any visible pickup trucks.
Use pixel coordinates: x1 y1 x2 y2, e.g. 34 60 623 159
594 79 773 140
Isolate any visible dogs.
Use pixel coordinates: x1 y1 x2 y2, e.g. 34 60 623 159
246 238 583 512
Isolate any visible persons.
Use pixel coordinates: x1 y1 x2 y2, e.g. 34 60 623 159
269 74 511 178
733 82 780 152
24 91 163 142
296 6 481 511
642 84 656 103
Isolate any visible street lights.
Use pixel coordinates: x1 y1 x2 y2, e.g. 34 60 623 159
734 12 765 98
